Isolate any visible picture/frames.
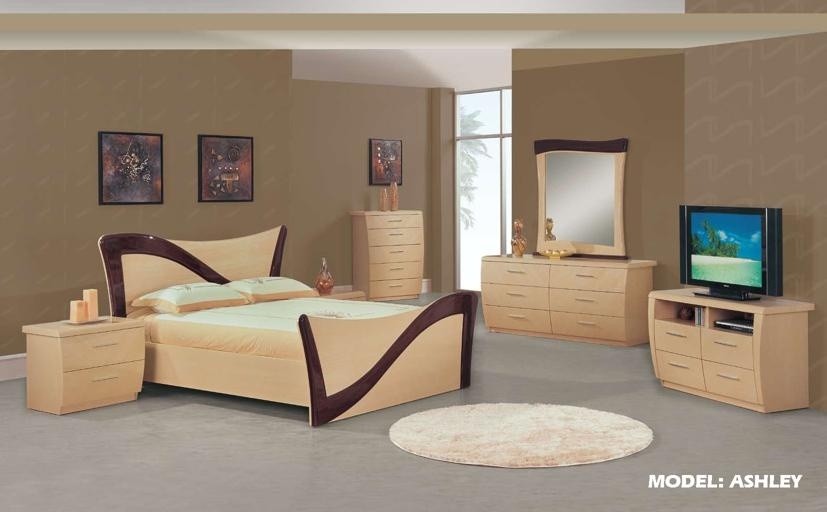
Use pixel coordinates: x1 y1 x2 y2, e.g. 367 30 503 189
96 130 254 206
368 138 402 187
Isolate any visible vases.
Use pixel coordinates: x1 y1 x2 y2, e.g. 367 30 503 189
313 255 335 297
510 217 527 258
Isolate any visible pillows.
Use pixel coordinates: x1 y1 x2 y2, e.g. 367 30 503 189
129 276 322 316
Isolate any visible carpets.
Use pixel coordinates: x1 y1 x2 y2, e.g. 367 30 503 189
386 401 656 470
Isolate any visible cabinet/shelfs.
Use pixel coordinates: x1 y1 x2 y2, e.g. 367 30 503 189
347 209 425 302
646 286 815 414
480 253 657 347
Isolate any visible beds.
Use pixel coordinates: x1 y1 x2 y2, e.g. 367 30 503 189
96 224 478 427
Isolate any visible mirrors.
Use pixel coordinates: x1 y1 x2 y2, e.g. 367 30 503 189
532 137 628 257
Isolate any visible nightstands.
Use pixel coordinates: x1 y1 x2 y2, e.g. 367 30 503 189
21 316 146 416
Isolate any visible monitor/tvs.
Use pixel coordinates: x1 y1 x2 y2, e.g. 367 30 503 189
678 203 783 301
